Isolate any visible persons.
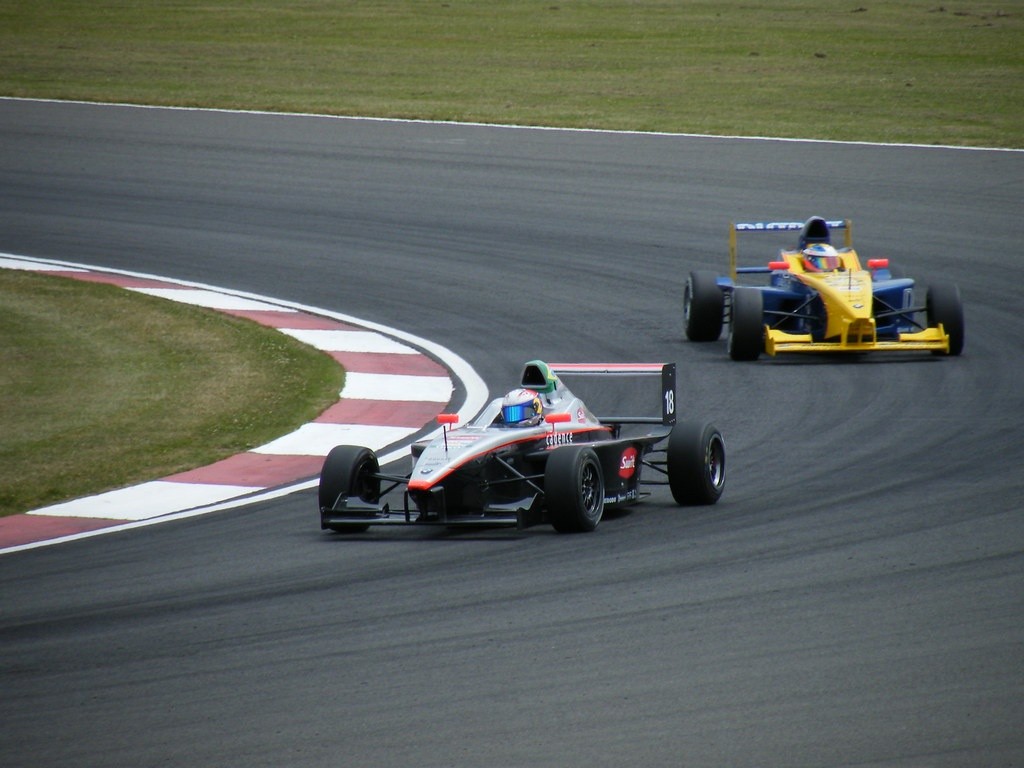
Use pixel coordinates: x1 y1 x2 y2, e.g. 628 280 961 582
499 389 543 427
803 243 840 272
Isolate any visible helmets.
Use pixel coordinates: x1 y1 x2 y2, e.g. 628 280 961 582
803 243 842 271
501 388 544 423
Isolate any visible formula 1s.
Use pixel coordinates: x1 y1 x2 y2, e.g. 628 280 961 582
318 360 728 533
683 215 967 358
